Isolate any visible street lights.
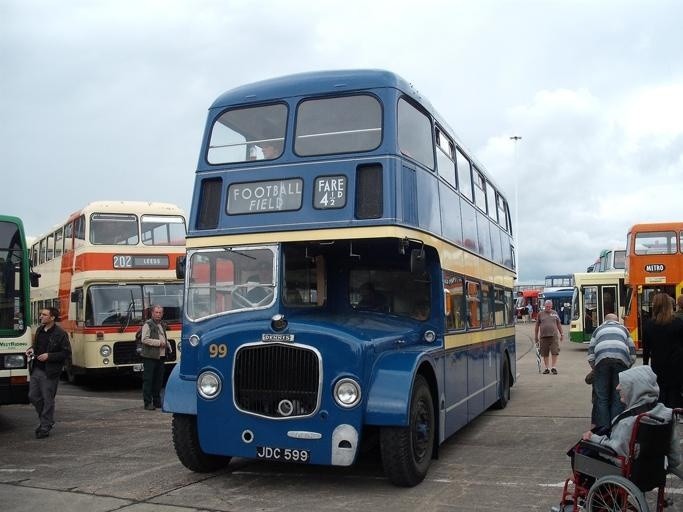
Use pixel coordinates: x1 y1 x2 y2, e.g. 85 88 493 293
509 135 521 286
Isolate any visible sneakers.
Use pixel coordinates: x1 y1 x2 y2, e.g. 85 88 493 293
34 422 51 439
543 369 550 375
153 397 163 408
143 401 155 410
551 369 558 375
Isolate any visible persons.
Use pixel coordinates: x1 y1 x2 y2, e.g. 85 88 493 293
260 136 284 159
135 303 171 409
562 303 569 325
25 307 72 438
551 365 673 512
640 292 682 423
535 299 564 375
587 313 636 430
354 281 389 312
676 294 682 315
526 300 533 318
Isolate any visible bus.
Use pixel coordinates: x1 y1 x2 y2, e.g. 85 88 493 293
0 213 40 403
515 221 683 355
27 199 189 386
159 69 516 487
153 237 234 316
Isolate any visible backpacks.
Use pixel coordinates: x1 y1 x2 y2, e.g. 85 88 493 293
135 322 155 353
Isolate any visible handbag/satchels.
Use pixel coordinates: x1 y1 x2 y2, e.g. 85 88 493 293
566 422 621 459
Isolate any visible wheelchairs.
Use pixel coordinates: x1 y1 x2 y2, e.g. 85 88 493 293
553 407 682 510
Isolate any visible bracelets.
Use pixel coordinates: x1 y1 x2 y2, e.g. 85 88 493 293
560 333 564 336
